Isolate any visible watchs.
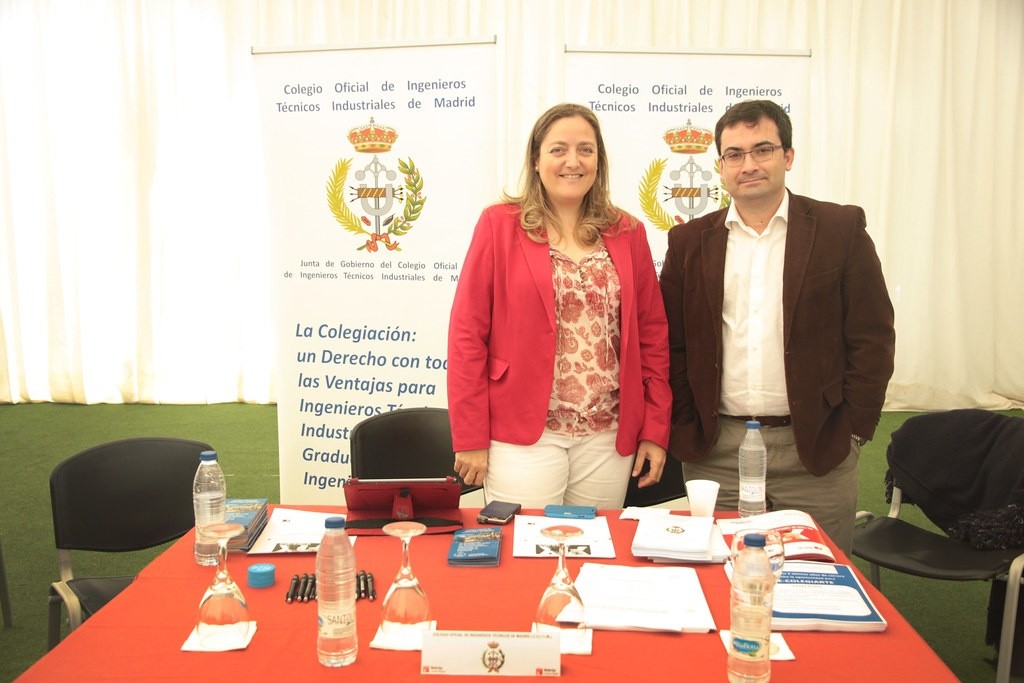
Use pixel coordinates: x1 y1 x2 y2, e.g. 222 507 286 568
851 432 868 447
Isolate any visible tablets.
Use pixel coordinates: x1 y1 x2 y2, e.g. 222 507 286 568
344 478 462 511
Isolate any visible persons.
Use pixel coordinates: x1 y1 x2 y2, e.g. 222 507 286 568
657 100 897 569
446 103 673 513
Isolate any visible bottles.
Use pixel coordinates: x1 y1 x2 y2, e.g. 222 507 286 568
728 534 770 683
193 449 227 566
315 516 358 667
736 420 767 518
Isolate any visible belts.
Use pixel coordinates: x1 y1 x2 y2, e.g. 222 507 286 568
721 414 792 428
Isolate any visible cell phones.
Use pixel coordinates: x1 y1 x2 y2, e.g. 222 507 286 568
544 505 596 519
477 500 521 524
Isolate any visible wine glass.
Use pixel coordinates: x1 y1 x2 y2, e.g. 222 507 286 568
380 519 430 647
198 522 250 644
532 524 589 653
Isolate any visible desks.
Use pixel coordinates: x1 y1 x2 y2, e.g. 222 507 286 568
11 496 959 682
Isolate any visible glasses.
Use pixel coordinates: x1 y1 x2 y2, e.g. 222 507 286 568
720 146 784 167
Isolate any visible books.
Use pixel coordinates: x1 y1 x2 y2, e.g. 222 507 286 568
203 492 893 678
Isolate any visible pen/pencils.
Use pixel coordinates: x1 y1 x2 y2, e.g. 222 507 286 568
285 569 376 601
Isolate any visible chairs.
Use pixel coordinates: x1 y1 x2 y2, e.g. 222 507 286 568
45 436 219 648
851 406 1024 681
346 407 487 510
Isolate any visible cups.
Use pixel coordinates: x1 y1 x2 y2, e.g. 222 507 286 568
685 477 721 519
732 528 785 591
